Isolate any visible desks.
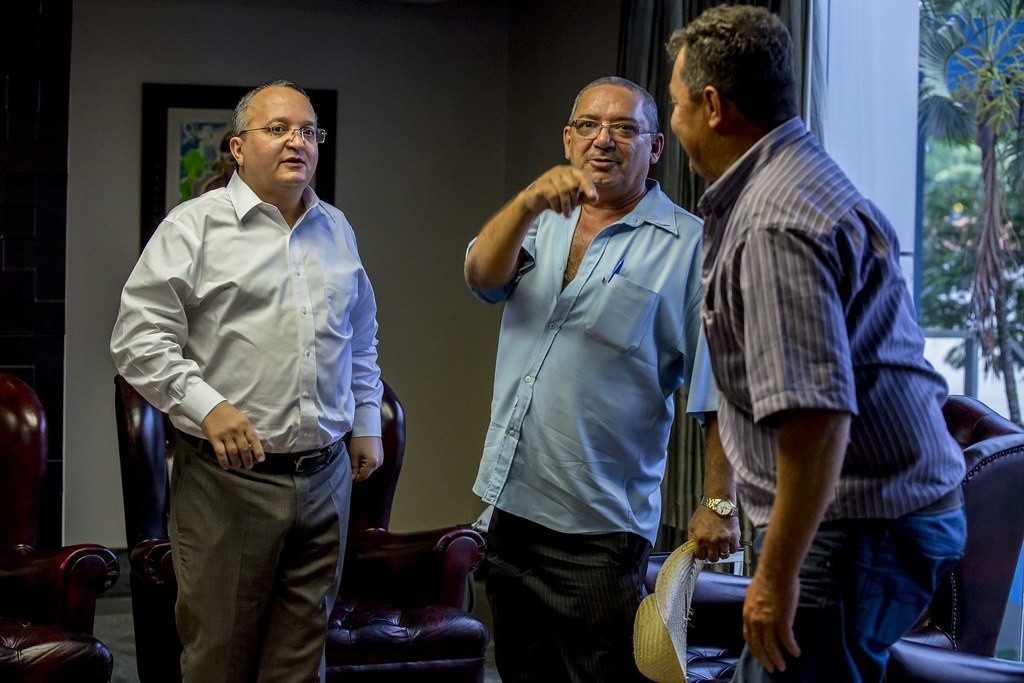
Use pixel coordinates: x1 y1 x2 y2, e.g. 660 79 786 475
649 525 745 576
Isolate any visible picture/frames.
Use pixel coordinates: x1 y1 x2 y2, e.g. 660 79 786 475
140 81 338 262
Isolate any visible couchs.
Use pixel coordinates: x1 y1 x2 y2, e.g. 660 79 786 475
896 394 1024 659
113 373 487 683
644 557 1024 683
0 373 120 683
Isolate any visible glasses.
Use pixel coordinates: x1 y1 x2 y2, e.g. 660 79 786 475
564 120 657 139
238 125 327 143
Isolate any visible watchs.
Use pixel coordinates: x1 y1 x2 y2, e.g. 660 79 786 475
700 496 739 520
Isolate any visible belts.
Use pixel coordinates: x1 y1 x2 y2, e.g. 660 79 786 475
173 427 342 474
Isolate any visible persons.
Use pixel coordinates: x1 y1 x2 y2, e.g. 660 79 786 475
464 77 741 683
666 4 969 683
109 79 385 683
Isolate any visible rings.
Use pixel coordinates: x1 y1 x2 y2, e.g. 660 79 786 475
719 550 730 556
